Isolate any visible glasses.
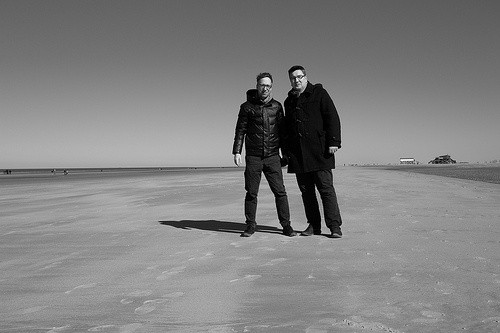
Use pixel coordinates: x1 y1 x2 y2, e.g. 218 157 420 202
290 74 305 80
258 83 272 88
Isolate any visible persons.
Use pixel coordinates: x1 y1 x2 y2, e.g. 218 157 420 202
232 72 297 236
284 66 341 238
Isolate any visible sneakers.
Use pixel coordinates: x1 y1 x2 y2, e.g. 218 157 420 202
283 226 296 236
331 226 342 237
243 225 254 237
301 224 321 235
